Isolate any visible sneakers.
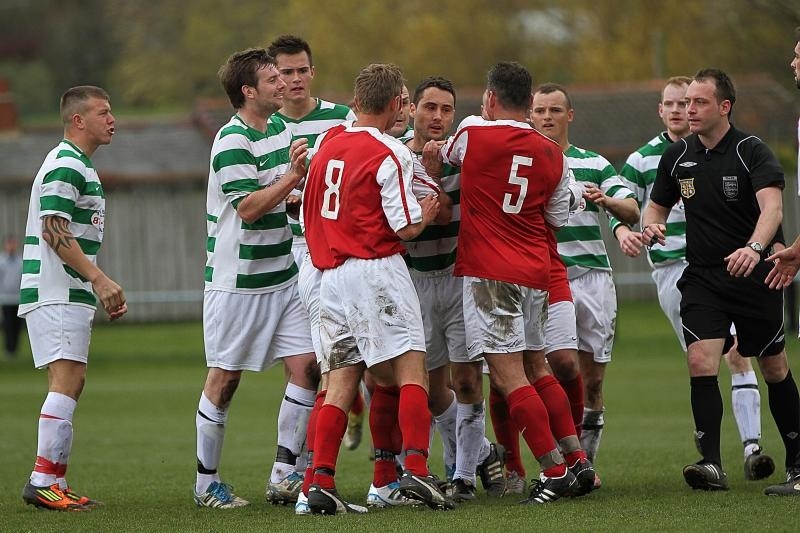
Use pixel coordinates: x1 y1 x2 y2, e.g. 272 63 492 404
193 394 800 515
58 484 105 510
22 479 92 513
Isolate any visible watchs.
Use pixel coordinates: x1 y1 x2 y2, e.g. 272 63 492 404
746 242 764 257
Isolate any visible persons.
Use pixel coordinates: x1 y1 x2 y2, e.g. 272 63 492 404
393 78 485 509
643 70 800 490
328 100 382 462
1 233 29 358
607 74 779 479
528 82 641 491
763 28 800 494
364 80 438 478
295 99 454 514
18 86 130 516
441 60 603 507
478 86 586 496
266 33 363 279
301 64 457 517
196 49 324 513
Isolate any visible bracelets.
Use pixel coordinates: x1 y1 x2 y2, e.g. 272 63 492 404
642 227 649 236
